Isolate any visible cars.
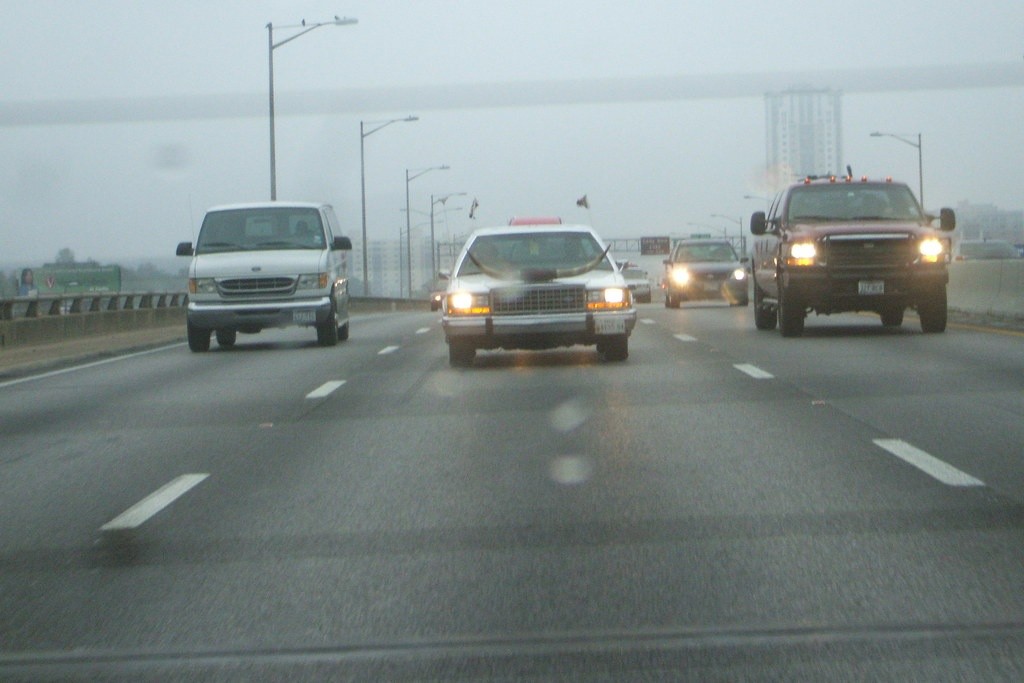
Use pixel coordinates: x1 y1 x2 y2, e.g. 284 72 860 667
618 267 652 304
437 223 638 365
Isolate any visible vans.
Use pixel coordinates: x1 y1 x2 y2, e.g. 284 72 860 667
508 215 562 225
173 201 355 352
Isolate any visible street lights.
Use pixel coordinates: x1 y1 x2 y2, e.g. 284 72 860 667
431 191 468 286
399 219 445 298
869 131 925 213
264 16 360 202
405 165 450 300
359 114 421 296
710 213 743 258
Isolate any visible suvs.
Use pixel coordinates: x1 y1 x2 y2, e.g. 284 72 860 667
747 173 957 339
660 236 740 308
